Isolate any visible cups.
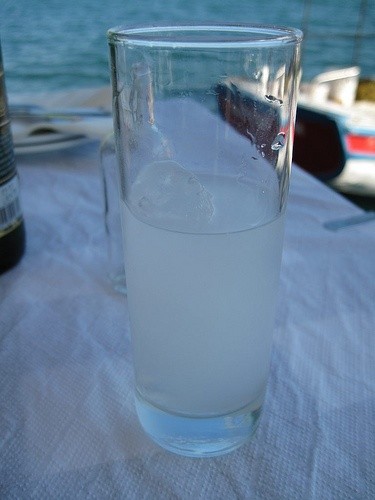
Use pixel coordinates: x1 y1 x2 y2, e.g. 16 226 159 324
107 19 304 459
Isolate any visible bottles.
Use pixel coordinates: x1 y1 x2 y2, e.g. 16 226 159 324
0 42 26 275
98 62 175 293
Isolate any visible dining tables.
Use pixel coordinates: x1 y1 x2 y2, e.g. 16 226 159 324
0 98 375 499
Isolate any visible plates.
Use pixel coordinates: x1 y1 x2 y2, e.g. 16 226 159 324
10 106 112 154
